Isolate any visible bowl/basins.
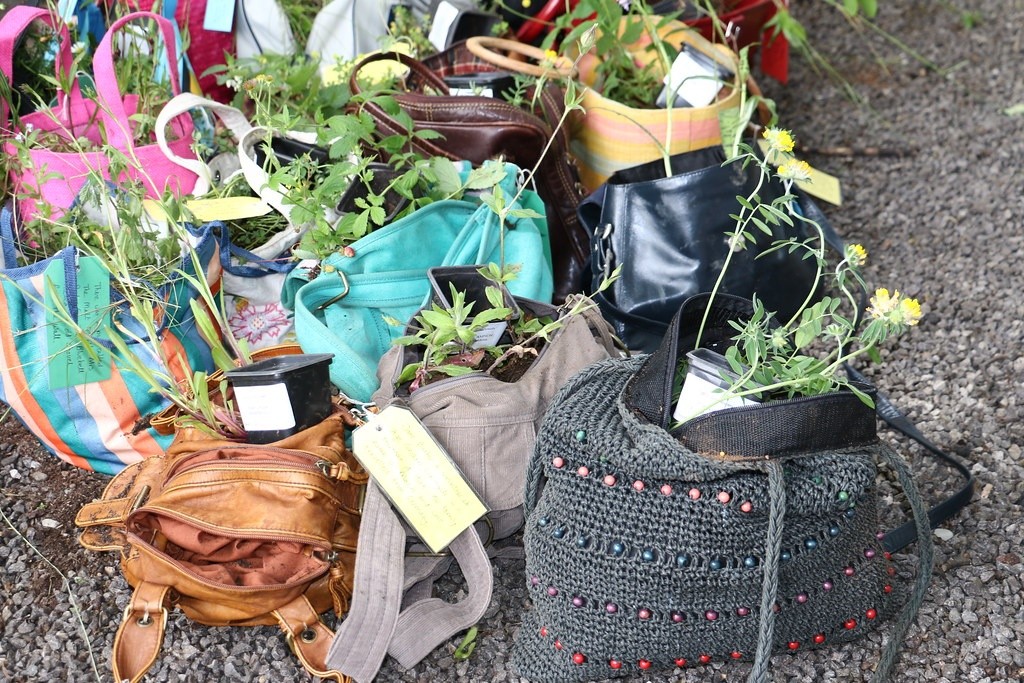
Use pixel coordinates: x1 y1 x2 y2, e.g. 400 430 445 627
224 353 335 444
428 265 521 327
672 348 782 423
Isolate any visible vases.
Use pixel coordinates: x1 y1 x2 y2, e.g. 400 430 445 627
224 353 337 441
253 134 329 200
669 347 768 425
444 70 516 105
657 42 731 110
333 161 409 226
425 0 498 52
426 265 522 349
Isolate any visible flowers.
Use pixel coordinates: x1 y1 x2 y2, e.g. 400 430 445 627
683 125 923 414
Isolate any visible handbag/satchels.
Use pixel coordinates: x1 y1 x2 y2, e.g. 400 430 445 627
0 0 768 473
324 262 623 682
75 347 368 683
507 295 934 683
577 142 828 360
